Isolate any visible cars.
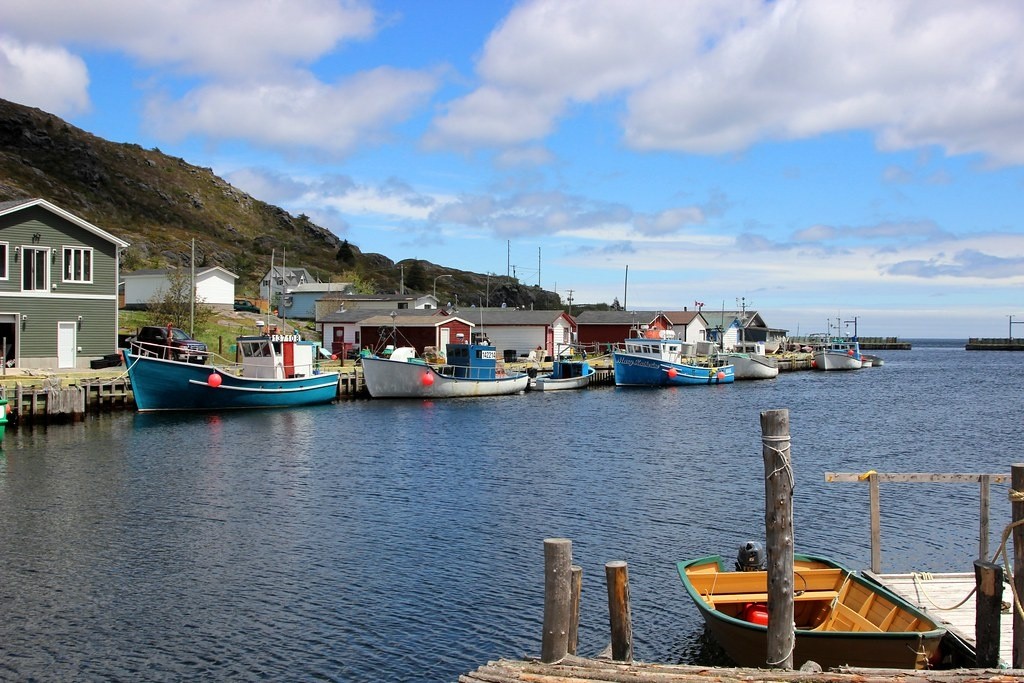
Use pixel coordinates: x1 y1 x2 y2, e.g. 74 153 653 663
234 299 260 314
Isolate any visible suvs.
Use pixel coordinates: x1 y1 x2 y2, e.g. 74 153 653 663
132 326 210 365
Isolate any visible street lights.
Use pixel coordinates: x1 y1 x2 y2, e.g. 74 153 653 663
434 275 451 308
1006 315 1015 344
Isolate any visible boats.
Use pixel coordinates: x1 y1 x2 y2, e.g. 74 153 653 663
123 247 341 413
0 399 9 442
536 343 596 391
812 310 884 370
707 297 779 378
358 297 528 396
613 309 734 386
676 541 946 668
